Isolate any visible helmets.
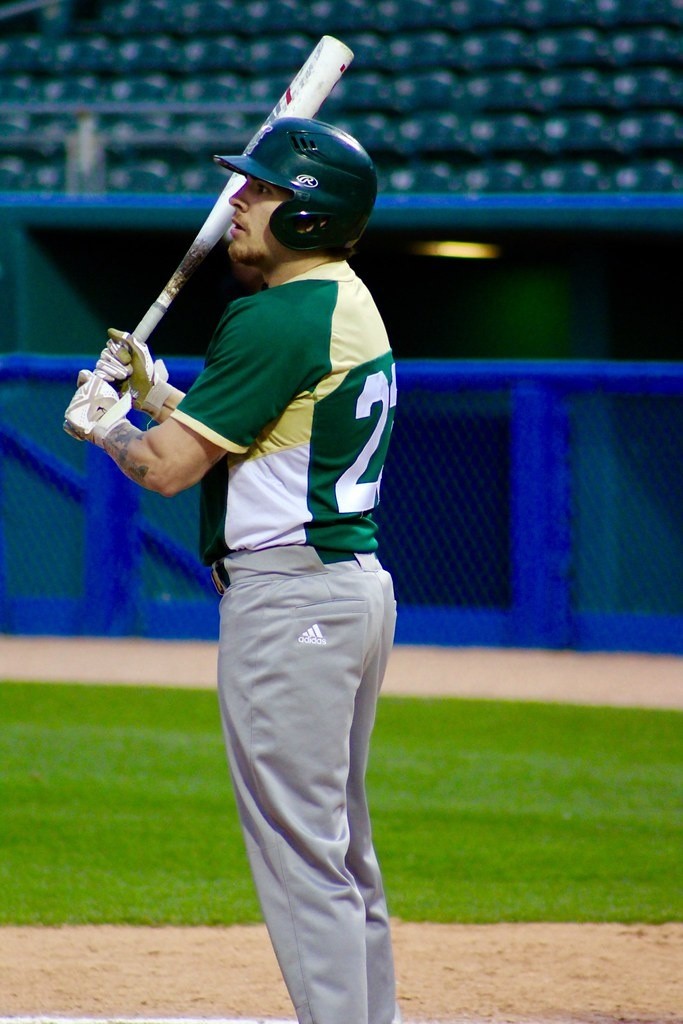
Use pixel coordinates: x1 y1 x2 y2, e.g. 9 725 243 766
215 117 377 250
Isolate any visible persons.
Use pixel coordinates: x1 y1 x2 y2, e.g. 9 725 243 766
63 116 397 1024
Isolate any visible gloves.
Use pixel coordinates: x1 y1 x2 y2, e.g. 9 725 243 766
92 327 172 420
64 368 133 448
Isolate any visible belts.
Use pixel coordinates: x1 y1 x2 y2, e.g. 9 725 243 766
210 546 378 593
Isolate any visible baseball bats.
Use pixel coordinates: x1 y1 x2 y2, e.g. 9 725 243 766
62 34 356 442
